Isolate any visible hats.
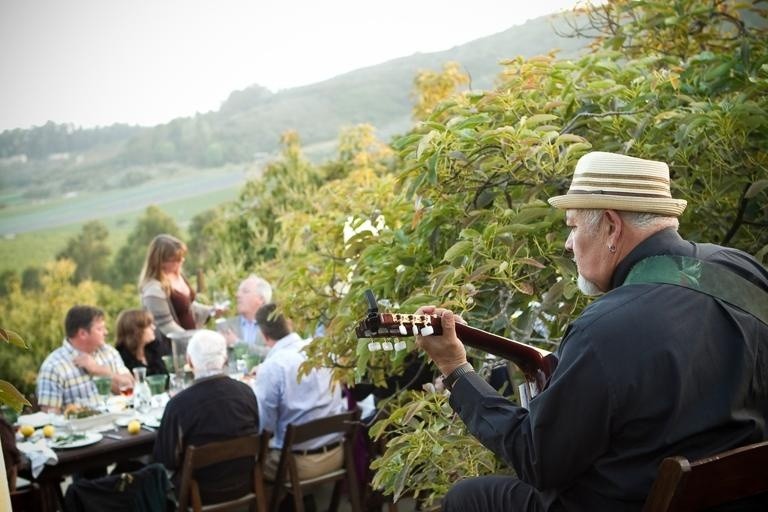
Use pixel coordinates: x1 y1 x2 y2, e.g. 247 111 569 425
548 151 687 216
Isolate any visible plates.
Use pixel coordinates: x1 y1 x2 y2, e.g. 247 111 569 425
17 410 51 429
46 430 104 450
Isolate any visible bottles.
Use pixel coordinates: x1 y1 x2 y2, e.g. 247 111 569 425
132 366 153 421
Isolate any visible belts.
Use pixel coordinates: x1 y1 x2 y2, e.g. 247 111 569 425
293 442 340 454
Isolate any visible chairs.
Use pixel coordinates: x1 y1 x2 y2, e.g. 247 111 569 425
641 438 768 512
62 462 169 511
262 406 361 510
169 427 276 510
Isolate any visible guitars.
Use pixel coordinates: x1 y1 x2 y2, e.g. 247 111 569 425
355 289 558 400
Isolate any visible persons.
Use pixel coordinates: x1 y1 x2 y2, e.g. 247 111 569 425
34 300 146 484
110 330 261 508
411 150 768 511
250 306 351 512
211 275 275 364
113 310 170 385
139 234 231 374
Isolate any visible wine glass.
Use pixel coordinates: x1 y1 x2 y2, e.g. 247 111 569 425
94 376 114 416
118 374 133 413
144 374 168 422
